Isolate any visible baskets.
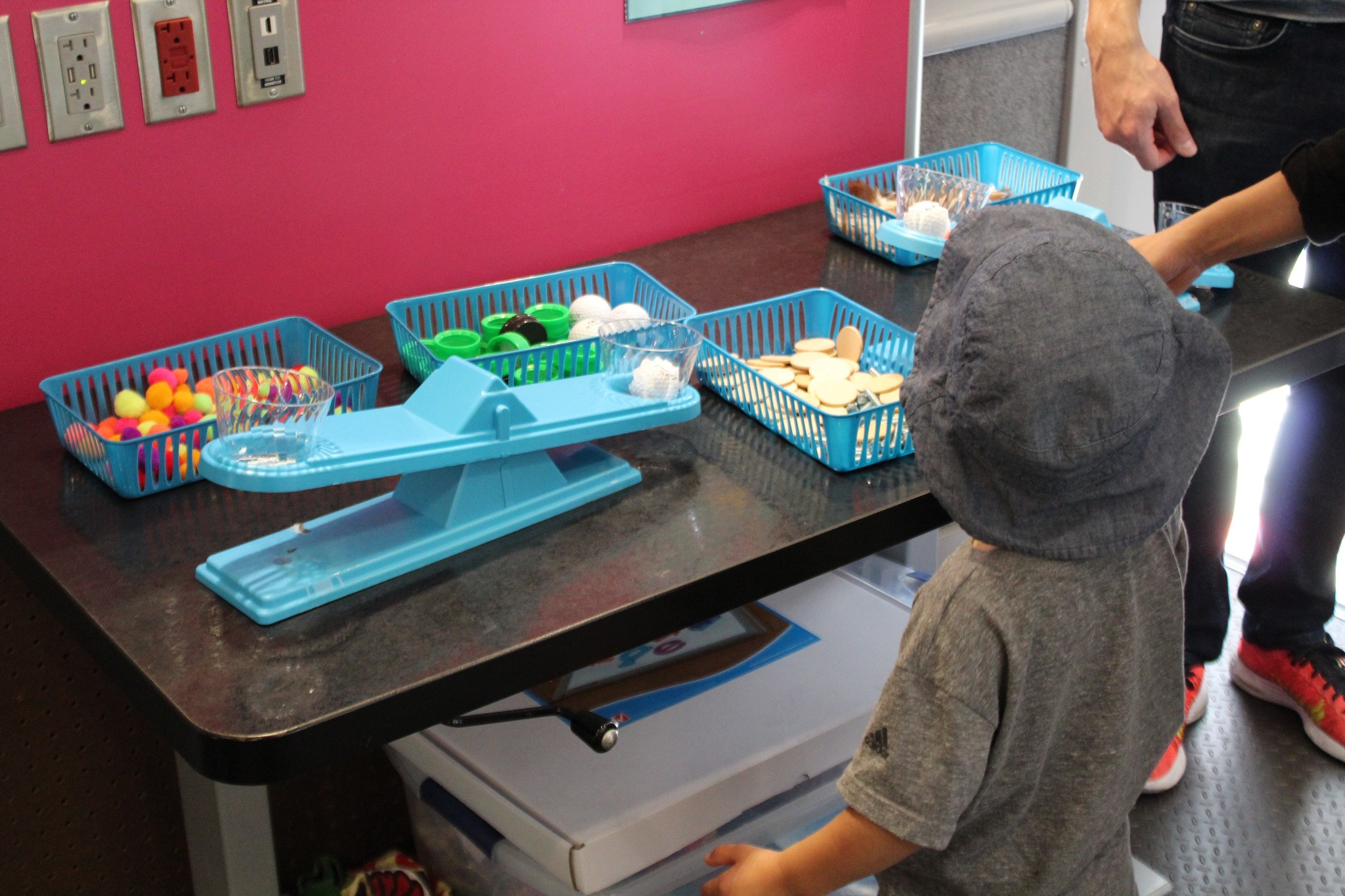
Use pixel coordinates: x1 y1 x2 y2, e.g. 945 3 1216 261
684 285 919 473
386 262 700 388
819 141 1082 267
39 318 383 497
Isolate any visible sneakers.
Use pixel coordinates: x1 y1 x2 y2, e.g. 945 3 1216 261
1230 631 1345 765
1143 664 1210 796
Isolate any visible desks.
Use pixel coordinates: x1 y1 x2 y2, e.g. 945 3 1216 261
0 200 1345 896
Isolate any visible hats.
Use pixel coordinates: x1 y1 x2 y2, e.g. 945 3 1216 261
900 200 1228 565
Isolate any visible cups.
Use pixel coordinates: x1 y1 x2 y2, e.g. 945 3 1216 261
1157 200 1204 233
597 317 704 400
210 365 335 466
895 164 994 241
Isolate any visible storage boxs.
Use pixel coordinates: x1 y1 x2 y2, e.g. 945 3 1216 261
384 553 933 896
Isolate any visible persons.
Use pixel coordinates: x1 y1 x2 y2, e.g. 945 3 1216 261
1085 0 1345 794
703 201 1233 896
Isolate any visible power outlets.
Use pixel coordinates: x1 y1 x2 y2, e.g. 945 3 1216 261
30 0 124 143
130 0 217 124
